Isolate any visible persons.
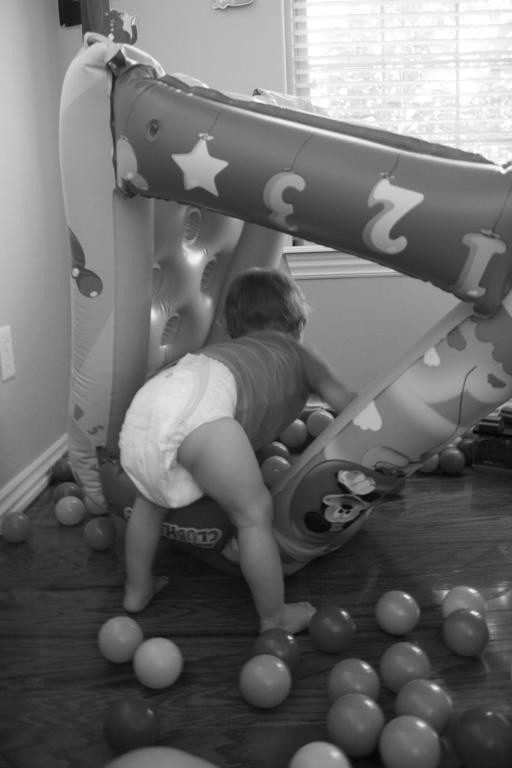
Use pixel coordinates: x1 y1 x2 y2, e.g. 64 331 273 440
102 747 222 768
117 266 359 637
